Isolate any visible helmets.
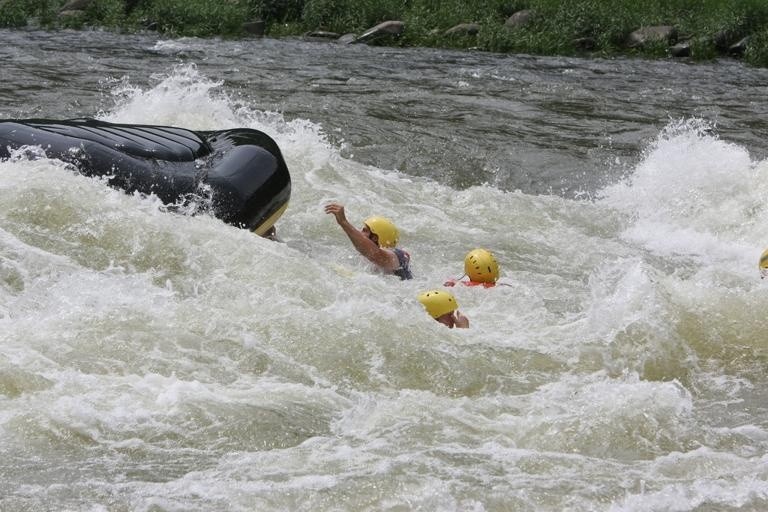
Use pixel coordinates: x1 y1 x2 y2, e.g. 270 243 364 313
364 215 400 248
464 249 500 283
417 290 458 319
759 247 768 270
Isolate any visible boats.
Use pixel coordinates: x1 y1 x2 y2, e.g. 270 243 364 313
1 118 293 238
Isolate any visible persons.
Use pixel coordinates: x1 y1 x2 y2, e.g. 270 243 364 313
417 289 469 328
324 202 413 280
443 248 500 288
